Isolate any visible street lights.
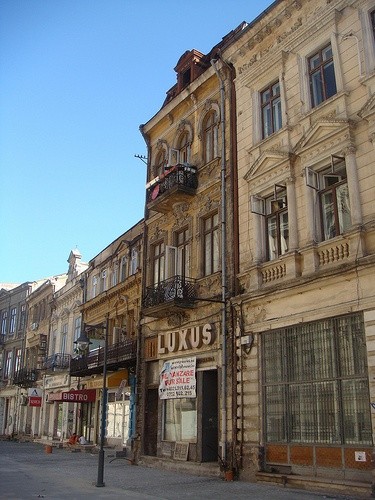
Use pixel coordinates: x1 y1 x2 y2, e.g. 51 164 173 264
73 312 110 487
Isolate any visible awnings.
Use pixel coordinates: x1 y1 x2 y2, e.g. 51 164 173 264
48 388 96 403
